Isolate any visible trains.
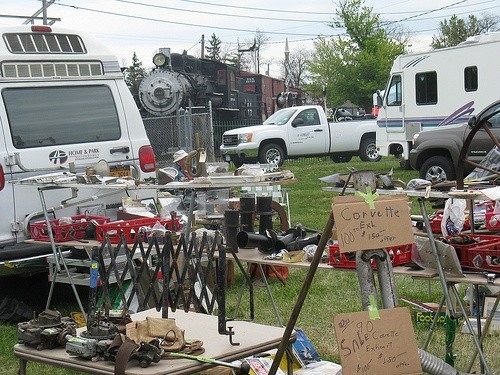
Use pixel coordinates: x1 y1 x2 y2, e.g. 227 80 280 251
137 48 305 125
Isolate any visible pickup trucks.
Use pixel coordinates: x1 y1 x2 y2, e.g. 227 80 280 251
219 104 382 169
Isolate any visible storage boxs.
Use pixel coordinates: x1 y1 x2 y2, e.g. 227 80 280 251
30 215 110 242
328 244 417 271
440 201 500 273
95 217 180 244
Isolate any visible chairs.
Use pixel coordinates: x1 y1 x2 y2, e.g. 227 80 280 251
241 162 291 228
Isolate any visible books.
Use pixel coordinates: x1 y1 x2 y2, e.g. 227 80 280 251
286 329 323 368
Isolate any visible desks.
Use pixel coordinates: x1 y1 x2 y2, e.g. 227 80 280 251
13 179 500 375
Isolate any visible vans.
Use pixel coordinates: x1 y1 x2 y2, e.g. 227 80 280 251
0 24 159 299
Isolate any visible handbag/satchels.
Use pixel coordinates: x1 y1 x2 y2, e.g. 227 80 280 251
106 317 186 375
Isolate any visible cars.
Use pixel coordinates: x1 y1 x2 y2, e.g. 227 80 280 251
410 99 500 191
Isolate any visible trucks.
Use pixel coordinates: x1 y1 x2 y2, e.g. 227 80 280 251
375 32 500 170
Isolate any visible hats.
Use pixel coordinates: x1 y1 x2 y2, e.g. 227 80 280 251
172 150 189 163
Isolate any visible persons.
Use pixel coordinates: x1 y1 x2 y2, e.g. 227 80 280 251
172 150 226 231
